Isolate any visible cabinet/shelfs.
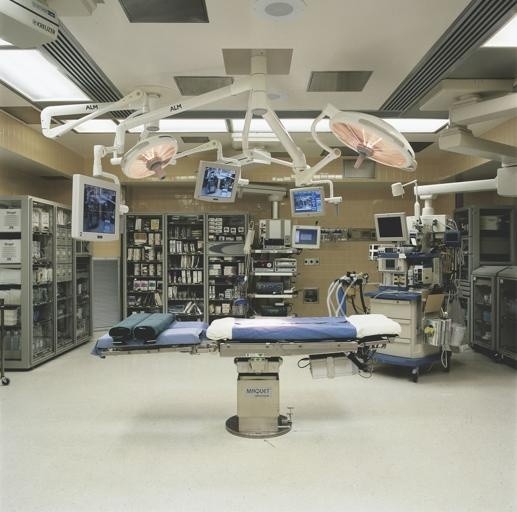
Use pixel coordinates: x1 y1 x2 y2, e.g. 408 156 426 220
1 195 92 372
116 210 250 323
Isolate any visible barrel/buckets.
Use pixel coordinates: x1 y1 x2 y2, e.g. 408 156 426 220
449 323 466 348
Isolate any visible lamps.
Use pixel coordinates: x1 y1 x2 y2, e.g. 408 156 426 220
310 102 419 171
120 128 178 180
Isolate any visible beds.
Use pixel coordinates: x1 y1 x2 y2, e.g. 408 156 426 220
90 314 402 436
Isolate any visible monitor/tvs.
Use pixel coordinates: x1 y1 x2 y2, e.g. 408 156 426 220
71 174 120 242
194 160 241 203
374 212 409 242
291 225 320 250
290 186 325 218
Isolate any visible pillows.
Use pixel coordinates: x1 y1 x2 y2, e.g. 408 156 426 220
108 313 176 342
